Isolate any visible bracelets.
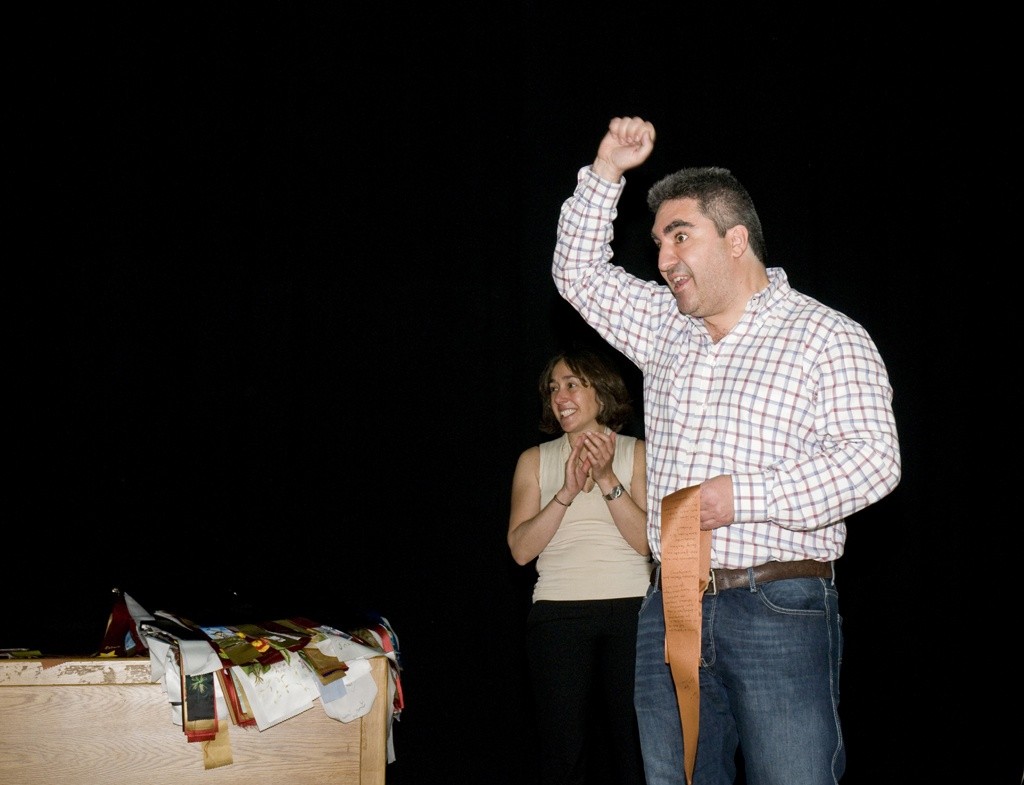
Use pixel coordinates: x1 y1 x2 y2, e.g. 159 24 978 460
554 495 572 507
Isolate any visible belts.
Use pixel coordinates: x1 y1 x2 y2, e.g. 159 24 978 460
649 559 833 595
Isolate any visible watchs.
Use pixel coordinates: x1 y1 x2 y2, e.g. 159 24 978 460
602 484 624 501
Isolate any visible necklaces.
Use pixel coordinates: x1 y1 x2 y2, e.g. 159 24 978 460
569 442 589 478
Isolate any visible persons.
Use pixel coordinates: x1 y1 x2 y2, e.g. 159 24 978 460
507 346 651 785
552 116 901 785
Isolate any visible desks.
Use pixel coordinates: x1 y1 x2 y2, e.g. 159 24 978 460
0 628 391 785
